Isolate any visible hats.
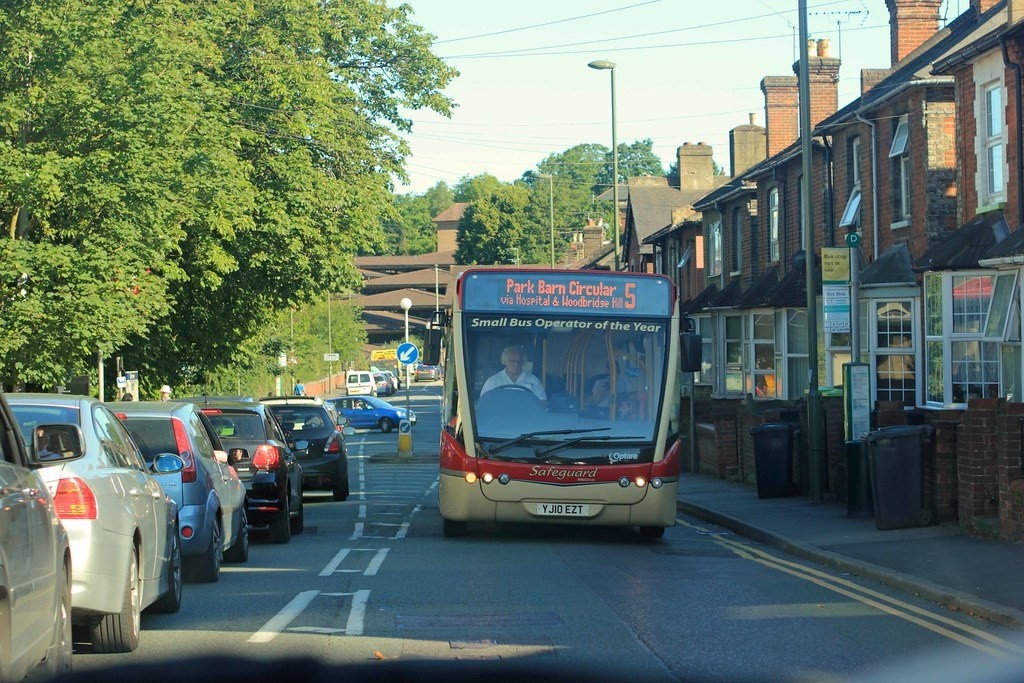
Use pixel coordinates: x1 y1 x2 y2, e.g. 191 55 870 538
159 385 171 393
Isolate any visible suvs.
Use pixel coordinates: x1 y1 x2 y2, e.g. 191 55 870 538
188 398 304 543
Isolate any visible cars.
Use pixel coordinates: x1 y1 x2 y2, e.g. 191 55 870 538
2 395 186 654
329 396 417 432
373 370 400 396
102 398 253 582
1 390 88 681
414 364 444 382
264 397 355 500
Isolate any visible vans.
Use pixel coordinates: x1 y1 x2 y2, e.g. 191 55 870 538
345 370 377 397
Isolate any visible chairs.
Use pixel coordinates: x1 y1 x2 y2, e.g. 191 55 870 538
521 359 608 401
335 401 343 408
303 414 325 429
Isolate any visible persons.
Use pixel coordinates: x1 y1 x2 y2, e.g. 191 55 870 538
37 427 61 460
354 400 363 410
294 379 305 396
122 393 134 402
231 449 243 462
480 344 547 408
592 360 637 420
159 385 172 402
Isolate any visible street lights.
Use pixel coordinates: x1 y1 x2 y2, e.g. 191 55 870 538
587 60 620 274
530 171 555 268
400 298 412 420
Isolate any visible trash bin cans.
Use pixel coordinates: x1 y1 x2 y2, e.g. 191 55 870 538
860 423 936 530
749 423 799 499
846 440 873 513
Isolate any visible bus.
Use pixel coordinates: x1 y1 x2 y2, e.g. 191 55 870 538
422 266 703 538
